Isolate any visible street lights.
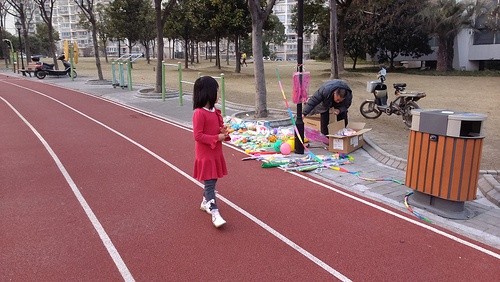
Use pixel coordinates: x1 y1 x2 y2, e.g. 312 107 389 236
14 19 26 76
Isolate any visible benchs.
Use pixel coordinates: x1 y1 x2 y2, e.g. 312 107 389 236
16 68 36 77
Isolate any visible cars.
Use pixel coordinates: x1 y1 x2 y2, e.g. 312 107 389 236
287 57 295 61
263 56 271 61
275 57 284 62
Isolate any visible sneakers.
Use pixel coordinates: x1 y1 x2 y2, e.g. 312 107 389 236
200 202 212 214
212 213 226 228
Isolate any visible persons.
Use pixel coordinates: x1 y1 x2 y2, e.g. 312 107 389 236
193 75 231 227
302 79 352 139
241 50 247 68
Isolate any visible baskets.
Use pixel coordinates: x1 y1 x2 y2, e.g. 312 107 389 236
367 81 385 93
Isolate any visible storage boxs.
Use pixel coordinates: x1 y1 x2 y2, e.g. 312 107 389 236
303 113 335 134
325 119 372 155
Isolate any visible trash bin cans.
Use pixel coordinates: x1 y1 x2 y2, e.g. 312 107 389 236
404 108 488 221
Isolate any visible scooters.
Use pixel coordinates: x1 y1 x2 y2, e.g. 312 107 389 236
360 66 427 128
31 51 77 79
